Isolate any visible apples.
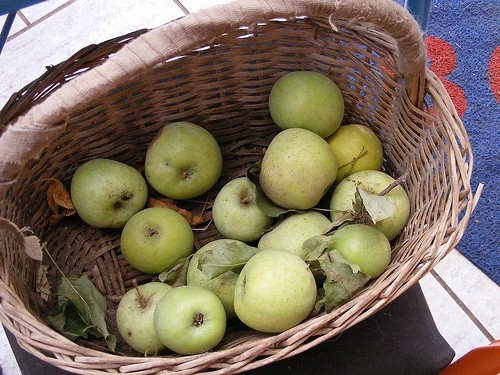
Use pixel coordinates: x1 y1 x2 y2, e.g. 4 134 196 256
329 170 411 242
234 249 316 333
144 122 222 199
120 207 194 274
268 71 345 138
326 123 383 183
326 224 391 280
116 281 178 356
255 211 333 262
259 128 337 211
154 286 226 355
70 158 147 229
212 177 280 243
186 238 251 317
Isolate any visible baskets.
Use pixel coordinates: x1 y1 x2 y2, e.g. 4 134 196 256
0 0 483 375
